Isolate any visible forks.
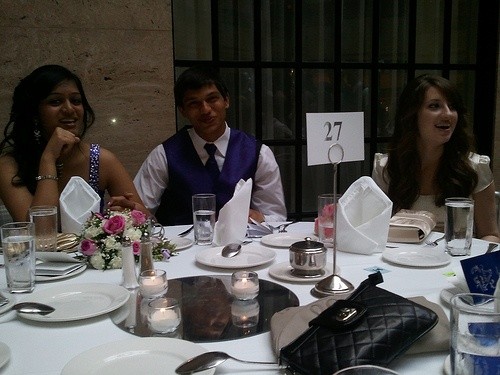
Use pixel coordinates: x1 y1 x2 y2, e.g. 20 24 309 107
424 235 445 246
279 222 292 233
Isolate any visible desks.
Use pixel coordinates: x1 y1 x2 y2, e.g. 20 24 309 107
0 221 500 375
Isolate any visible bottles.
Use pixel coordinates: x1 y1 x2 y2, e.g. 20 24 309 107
139 232 155 277
120 236 140 288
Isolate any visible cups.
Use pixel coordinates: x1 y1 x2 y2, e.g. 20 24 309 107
0 222 36 293
29 205 58 252
449 293 500 375
231 270 259 300
444 197 474 256
192 194 216 246
148 299 182 333
139 269 168 299
317 194 342 247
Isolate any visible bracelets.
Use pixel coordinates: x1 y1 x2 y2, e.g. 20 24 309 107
36 175 59 181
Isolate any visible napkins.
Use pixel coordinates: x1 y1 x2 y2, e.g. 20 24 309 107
59 176 101 237
335 175 393 256
212 177 253 247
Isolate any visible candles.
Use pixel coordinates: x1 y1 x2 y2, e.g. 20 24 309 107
142 272 165 296
233 278 256 295
152 306 177 332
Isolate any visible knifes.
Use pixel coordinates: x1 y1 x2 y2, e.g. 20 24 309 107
179 225 194 235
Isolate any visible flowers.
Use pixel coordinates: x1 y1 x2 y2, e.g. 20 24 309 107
70 207 179 272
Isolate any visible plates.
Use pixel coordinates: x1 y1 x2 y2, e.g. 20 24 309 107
16 282 130 322
36 264 87 281
0 342 11 368
440 287 500 315
167 233 341 282
444 354 451 375
0 291 16 314
382 247 451 267
60 337 216 375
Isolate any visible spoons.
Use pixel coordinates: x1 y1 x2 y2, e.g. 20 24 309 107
0 302 55 316
222 240 253 258
175 351 279 375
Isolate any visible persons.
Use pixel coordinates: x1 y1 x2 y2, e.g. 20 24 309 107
133 68 287 227
372 74 500 244
0 64 158 235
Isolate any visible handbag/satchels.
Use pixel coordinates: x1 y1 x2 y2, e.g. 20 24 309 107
279 271 439 375
270 295 451 363
5 232 80 256
387 208 437 243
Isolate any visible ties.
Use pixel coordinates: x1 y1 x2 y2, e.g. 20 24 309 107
204 143 221 182
